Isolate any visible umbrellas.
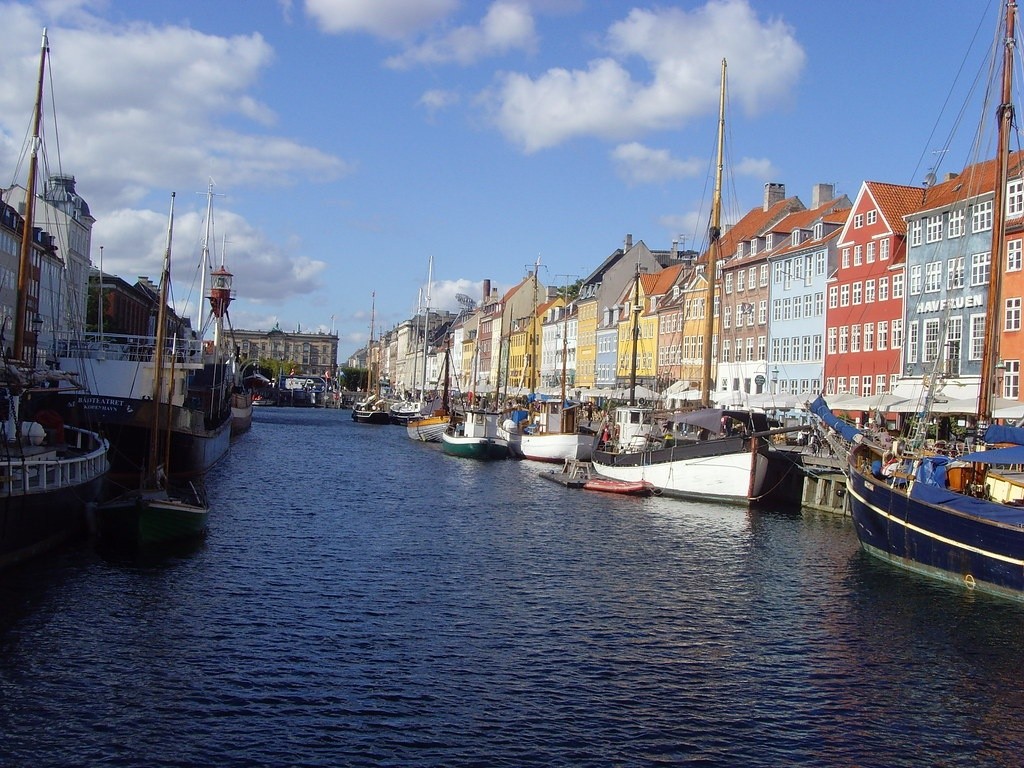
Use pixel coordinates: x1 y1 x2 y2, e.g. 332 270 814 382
415 385 1024 419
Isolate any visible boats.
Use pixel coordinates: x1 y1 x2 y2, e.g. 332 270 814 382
251 400 274 406
808 394 1024 603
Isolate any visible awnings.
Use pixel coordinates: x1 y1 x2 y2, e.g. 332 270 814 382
892 378 981 400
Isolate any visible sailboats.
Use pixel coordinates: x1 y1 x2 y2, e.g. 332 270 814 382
0 64 260 564
352 139 812 513
